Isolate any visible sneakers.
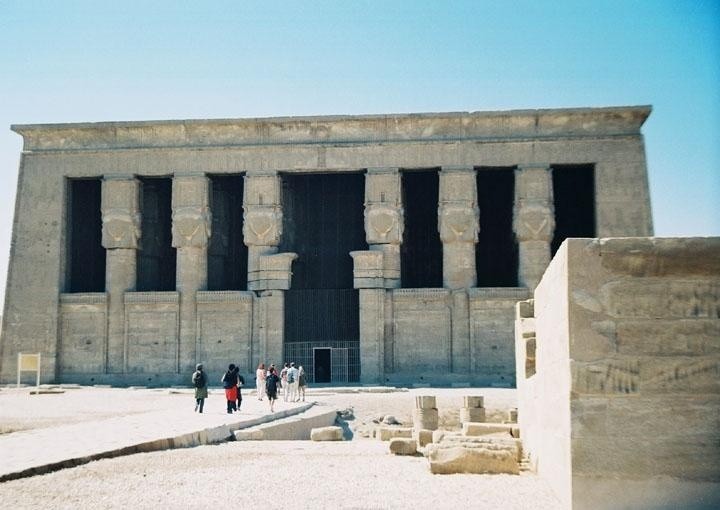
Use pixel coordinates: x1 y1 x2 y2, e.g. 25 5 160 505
194 405 198 412
199 410 202 413
228 407 241 414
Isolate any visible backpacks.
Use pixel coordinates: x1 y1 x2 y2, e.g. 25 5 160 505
194 371 205 388
223 370 236 389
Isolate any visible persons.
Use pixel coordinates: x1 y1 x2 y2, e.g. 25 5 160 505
256 361 307 403
192 363 208 413
220 363 241 414
236 375 244 409
265 368 278 414
